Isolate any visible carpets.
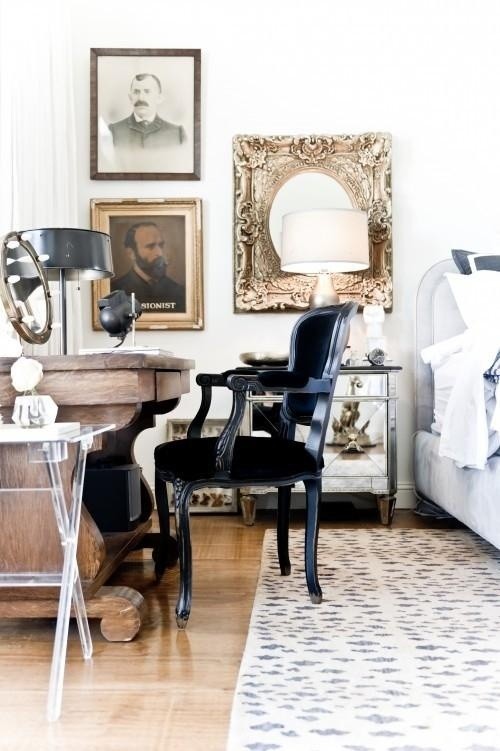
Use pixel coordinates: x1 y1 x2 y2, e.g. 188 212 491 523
218 520 500 740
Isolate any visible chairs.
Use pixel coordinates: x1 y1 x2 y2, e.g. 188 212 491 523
137 297 361 633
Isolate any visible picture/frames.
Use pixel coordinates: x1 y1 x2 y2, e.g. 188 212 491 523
87 47 200 181
88 197 204 333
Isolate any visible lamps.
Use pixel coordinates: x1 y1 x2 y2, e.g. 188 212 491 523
271 206 372 312
8 225 121 360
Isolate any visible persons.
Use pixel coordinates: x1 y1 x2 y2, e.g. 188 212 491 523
109 73 188 154
110 221 185 314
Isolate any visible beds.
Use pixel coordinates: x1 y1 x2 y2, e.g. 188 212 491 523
404 240 500 554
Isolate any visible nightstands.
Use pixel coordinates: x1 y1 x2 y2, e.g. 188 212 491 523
230 356 394 527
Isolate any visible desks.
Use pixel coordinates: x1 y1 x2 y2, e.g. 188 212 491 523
2 346 200 649
0 412 118 729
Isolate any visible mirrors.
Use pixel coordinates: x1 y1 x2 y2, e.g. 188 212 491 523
233 130 396 320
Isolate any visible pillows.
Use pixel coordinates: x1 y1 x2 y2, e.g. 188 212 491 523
443 266 500 363
444 246 500 274
